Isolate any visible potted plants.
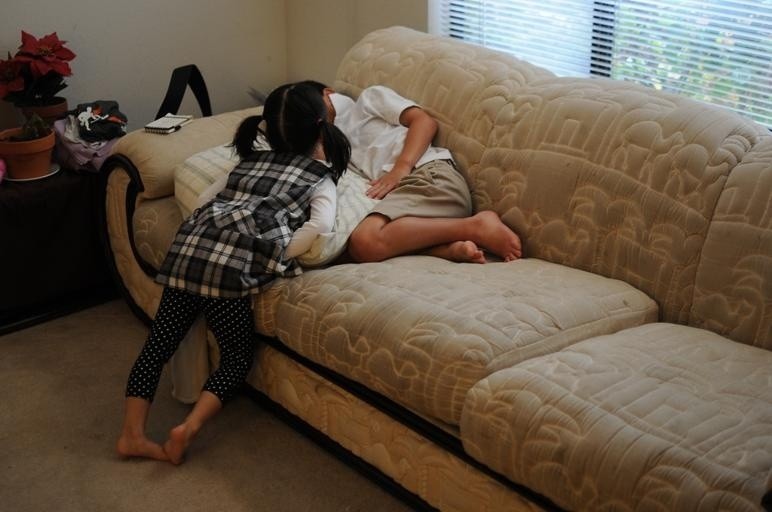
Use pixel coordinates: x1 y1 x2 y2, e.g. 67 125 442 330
1 113 56 182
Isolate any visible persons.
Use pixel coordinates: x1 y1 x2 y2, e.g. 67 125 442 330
117 78 356 467
298 79 525 267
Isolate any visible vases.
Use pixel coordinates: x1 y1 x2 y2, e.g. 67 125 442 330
19 97 69 125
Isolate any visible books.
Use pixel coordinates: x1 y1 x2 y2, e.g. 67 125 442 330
144 113 193 135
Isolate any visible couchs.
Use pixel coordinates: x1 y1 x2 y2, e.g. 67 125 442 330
93 25 772 512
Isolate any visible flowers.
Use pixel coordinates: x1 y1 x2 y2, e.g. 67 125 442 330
0 30 77 103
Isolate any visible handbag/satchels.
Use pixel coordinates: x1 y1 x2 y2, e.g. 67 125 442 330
76 101 124 142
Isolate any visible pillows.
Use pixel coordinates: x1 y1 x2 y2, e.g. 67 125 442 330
171 131 386 269
